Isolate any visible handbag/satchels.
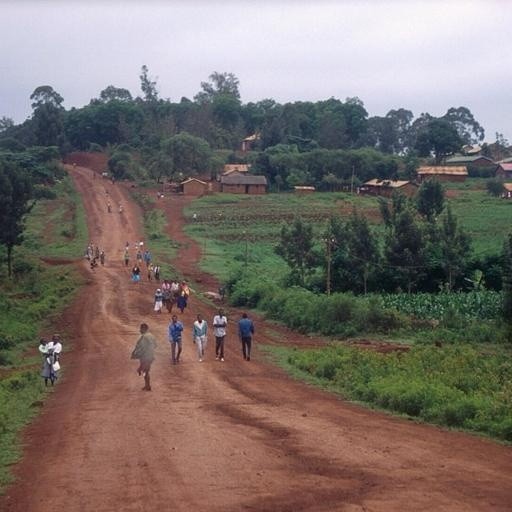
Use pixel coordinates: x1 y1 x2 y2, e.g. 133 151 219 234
185 292 189 298
52 360 60 371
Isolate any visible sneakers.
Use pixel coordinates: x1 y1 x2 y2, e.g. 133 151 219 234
243 355 250 361
173 358 179 364
216 354 224 361
198 358 203 362
142 386 151 391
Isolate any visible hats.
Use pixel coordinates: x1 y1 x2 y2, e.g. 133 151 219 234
157 288 160 291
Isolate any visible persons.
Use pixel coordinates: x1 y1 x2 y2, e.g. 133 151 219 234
86 240 105 269
38 337 63 386
45 334 62 371
213 309 227 362
192 212 197 222
157 191 160 200
130 323 158 391
93 171 123 213
168 315 183 365
192 314 208 362
238 313 254 361
124 239 190 313
